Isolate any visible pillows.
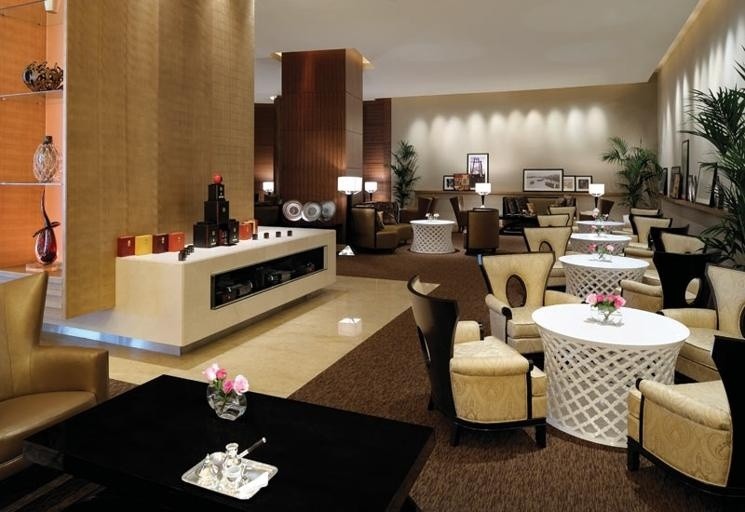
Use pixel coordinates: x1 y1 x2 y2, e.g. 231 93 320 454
261 200 273 206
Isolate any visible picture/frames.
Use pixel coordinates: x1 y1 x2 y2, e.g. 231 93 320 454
658 138 721 208
467 153 489 191
442 175 454 191
563 175 576 193
523 169 563 192
575 175 593 192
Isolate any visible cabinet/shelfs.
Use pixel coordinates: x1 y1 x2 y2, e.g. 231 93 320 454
114 226 337 357
0 0 129 319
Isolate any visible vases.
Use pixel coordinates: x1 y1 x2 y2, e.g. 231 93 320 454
206 384 247 421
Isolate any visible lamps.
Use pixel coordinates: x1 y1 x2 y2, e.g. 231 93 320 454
589 183 605 207
364 181 377 201
262 181 274 195
475 182 491 208
337 176 363 256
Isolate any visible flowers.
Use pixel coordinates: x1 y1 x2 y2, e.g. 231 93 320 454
201 363 250 414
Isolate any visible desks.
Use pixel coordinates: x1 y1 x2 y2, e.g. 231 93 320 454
23 374 438 510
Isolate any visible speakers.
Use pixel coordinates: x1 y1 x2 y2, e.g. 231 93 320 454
193 184 239 247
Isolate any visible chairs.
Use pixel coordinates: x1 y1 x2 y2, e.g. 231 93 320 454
0 270 109 481
254 205 279 228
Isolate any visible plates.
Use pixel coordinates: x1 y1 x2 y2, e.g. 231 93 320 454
282 200 336 222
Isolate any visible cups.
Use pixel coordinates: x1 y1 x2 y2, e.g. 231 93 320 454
252 230 293 240
199 442 250 492
178 245 194 261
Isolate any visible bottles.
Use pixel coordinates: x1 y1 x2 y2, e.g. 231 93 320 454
32 136 61 183
